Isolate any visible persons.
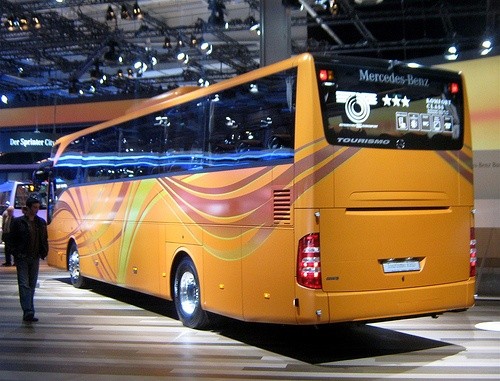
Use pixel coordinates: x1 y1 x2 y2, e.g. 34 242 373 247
10 196 49 321
22 206 29 216
2 205 17 267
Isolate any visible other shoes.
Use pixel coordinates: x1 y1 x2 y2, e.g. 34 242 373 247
24 313 39 321
13 262 16 265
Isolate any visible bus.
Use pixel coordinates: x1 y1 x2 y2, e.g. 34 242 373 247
33 50 478 329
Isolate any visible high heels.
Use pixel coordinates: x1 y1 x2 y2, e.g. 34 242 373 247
2 261 11 265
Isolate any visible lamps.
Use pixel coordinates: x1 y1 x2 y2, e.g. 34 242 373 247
1 92 12 106
4 15 41 33
68 31 214 96
208 1 228 29
249 1 260 35
479 30 492 49
105 1 142 21
447 32 460 55
123 79 282 154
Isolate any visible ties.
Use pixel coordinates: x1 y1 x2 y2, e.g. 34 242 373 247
5 216 11 232
